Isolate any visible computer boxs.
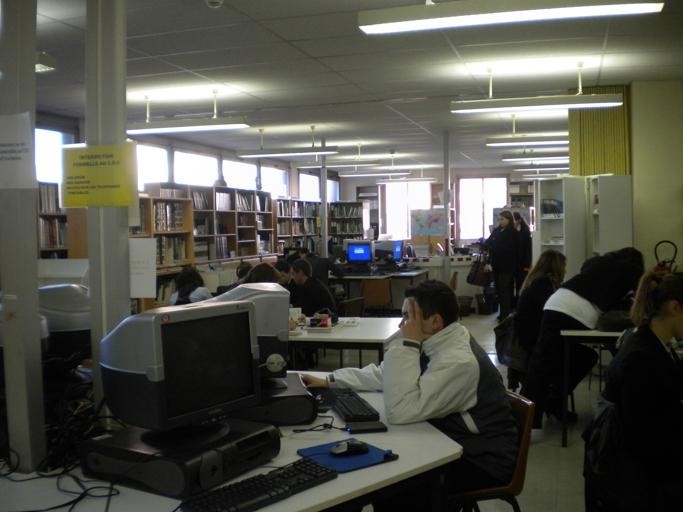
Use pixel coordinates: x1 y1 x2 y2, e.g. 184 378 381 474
81 418 280 501
229 373 317 426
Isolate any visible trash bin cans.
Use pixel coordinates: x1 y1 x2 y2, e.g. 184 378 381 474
0 283 93 459
475 293 498 314
456 296 473 316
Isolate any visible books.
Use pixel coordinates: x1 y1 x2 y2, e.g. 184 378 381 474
129 189 362 304
37 184 69 248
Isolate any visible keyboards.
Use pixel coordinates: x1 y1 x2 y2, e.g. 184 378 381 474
180 456 337 512
399 265 421 272
333 388 379 422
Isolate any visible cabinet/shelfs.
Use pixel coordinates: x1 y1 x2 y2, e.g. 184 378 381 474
499 171 634 293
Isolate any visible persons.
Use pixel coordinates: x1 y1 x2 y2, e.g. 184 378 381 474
481 211 520 322
519 246 645 429
169 248 345 370
511 211 532 296
515 249 599 427
602 270 683 511
301 280 519 510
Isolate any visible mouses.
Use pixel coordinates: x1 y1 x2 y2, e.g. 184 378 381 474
330 439 369 456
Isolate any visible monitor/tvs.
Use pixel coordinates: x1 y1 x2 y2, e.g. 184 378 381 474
342 238 405 265
36 283 91 368
199 282 291 391
99 301 263 449
